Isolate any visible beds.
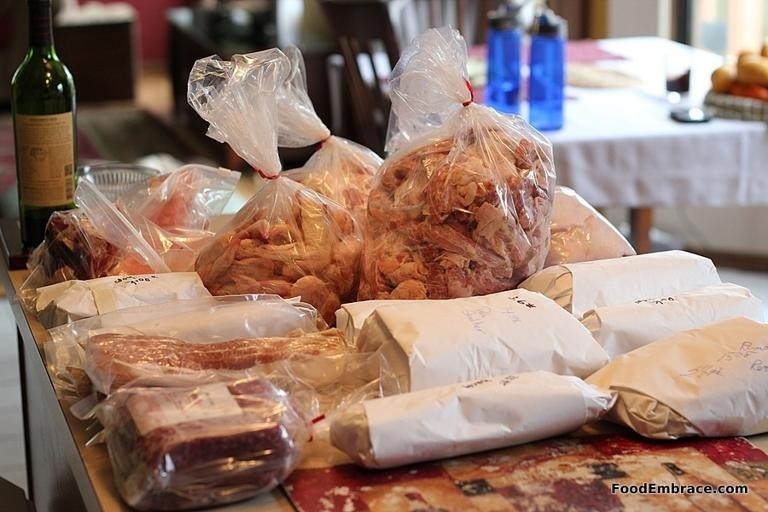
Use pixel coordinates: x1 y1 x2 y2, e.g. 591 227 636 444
356 34 768 255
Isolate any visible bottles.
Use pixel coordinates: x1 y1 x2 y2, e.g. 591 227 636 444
485 16 521 116
526 11 566 131
11 1 82 255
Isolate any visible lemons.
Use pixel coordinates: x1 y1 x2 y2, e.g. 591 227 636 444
737 43 768 82
711 67 731 94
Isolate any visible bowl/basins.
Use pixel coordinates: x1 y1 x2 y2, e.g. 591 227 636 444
76 164 162 200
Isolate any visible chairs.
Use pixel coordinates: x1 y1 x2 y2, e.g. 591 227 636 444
314 2 401 161
